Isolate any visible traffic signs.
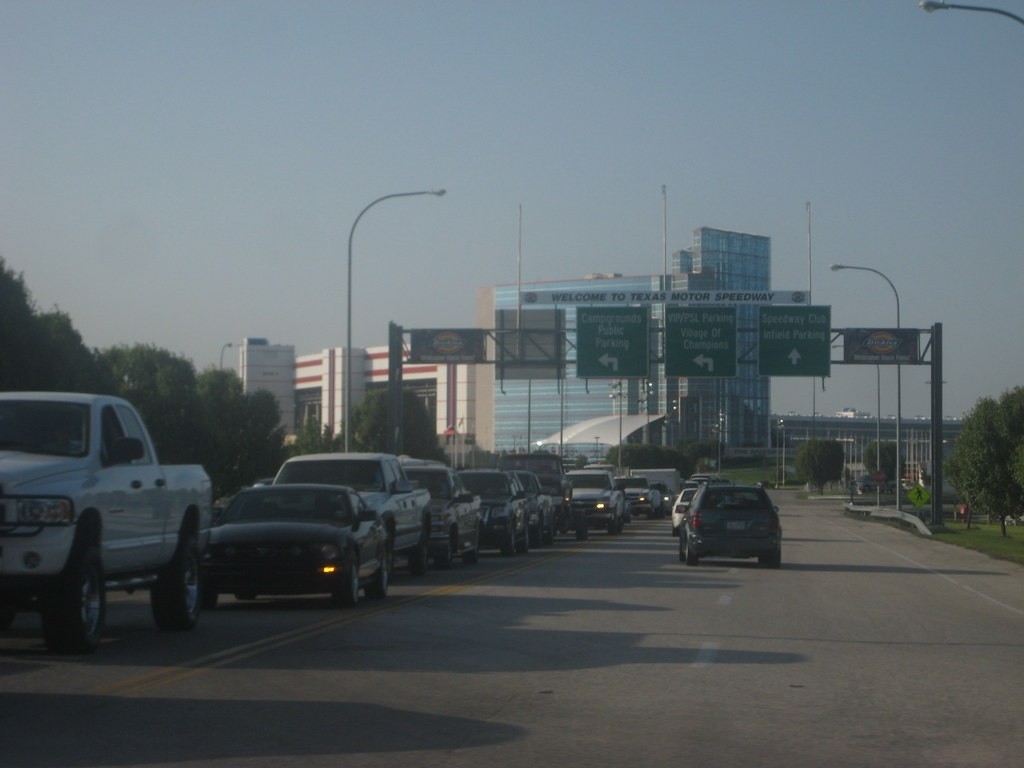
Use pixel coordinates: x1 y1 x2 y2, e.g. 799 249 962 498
761 305 832 378
665 304 736 378
577 304 652 379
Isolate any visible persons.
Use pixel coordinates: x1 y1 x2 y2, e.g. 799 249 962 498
314 493 337 520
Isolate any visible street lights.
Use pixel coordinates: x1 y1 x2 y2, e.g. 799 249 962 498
831 263 902 512
644 380 655 443
218 343 232 371
718 410 724 473
609 379 622 469
345 188 448 452
777 417 787 489
671 399 679 447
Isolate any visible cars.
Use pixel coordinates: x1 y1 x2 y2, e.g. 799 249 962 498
651 474 735 519
456 470 532 558
672 487 698 536
204 484 391 610
516 471 556 547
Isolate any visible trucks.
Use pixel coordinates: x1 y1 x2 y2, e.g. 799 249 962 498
495 453 574 537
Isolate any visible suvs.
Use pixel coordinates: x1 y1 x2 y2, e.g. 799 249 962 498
567 470 627 538
614 476 662 524
679 479 783 567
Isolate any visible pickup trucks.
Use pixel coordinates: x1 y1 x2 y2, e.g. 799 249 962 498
272 453 434 578
396 456 482 569
0 393 214 657
